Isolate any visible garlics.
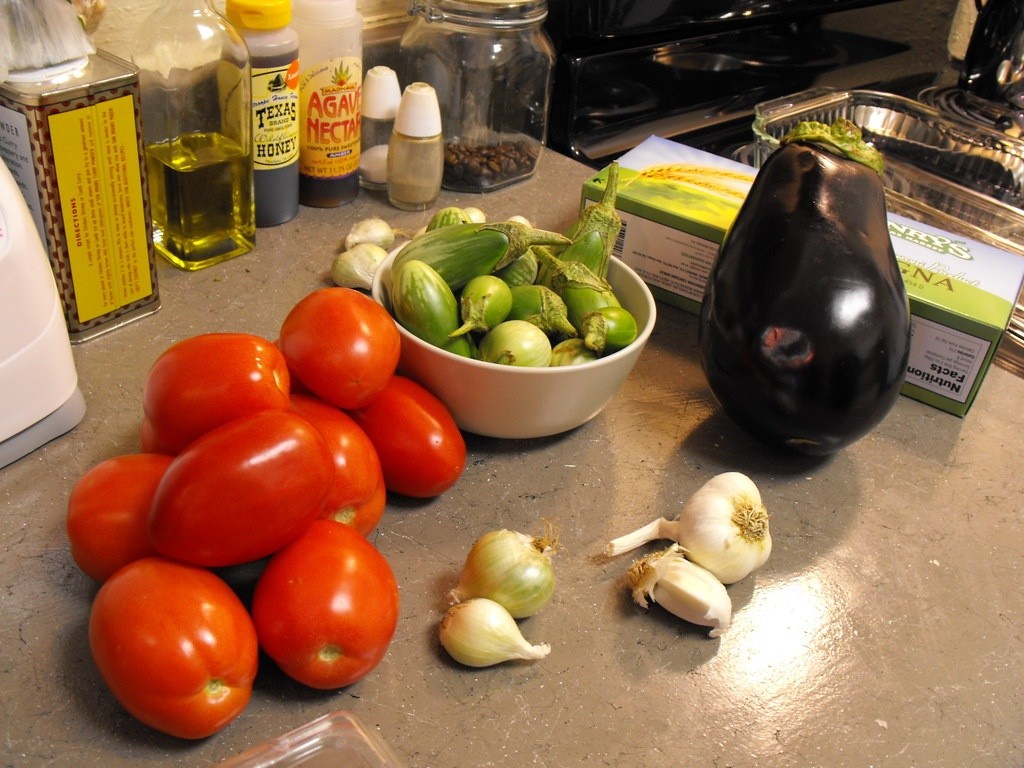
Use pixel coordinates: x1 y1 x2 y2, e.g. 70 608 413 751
332 207 537 290
439 529 556 668
645 473 772 629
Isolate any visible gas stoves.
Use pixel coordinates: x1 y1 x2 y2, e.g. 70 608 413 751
551 22 920 165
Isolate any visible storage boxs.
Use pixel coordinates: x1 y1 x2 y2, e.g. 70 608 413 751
577 133 1024 421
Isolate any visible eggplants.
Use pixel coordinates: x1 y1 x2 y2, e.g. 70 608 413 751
389 204 637 366
697 121 912 458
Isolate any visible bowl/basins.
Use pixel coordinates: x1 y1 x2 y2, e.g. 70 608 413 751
371 230 657 439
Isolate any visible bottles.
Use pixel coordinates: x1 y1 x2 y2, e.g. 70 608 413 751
398 1 554 193
293 1 361 208
388 81 443 211
130 1 256 271
360 66 401 191
225 1 299 227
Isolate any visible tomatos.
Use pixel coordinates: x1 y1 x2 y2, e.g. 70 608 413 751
67 287 465 740
